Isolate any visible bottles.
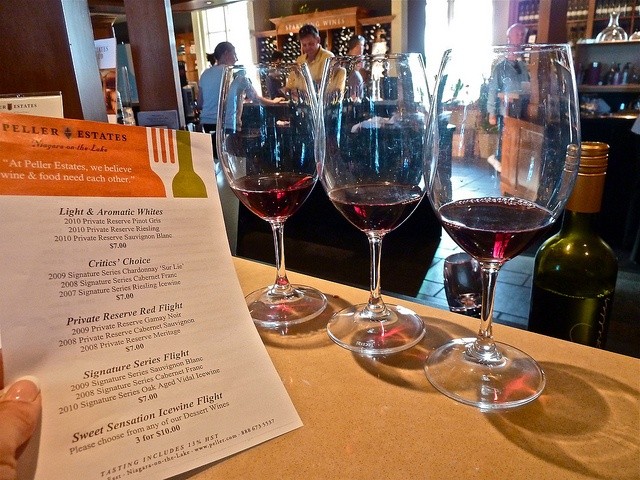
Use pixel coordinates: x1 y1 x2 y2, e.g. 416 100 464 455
587 60 639 85
527 141 619 351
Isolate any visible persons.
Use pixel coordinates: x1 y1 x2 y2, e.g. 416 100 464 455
0 347 42 480
288 25 344 104
197 40 286 156
486 23 534 174
264 49 285 101
347 33 368 103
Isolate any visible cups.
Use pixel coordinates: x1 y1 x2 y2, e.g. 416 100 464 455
443 251 481 319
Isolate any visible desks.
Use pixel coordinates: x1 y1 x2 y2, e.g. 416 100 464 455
179 244 640 478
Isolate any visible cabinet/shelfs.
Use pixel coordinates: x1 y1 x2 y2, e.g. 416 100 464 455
252 4 399 102
506 2 639 95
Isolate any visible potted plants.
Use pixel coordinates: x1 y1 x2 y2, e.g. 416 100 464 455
443 80 469 158
476 76 501 159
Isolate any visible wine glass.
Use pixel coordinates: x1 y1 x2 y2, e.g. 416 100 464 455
215 60 329 328
422 42 582 411
313 52 440 356
